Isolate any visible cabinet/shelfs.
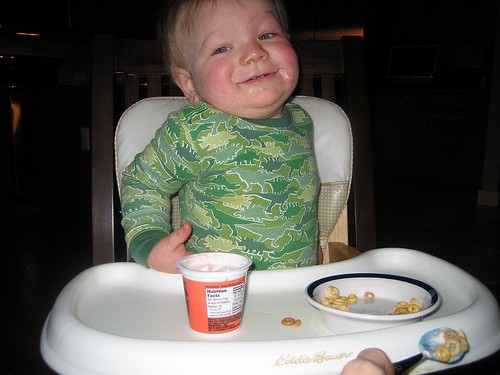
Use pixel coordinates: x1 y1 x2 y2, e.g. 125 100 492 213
374 89 489 184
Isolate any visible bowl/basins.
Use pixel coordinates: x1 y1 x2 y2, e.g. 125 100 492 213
304 273 442 334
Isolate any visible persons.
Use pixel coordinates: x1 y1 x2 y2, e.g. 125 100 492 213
121 0 324 274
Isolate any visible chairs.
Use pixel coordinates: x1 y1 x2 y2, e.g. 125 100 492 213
41 32 500 375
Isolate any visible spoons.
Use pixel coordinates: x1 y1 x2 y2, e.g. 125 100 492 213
392 327 465 375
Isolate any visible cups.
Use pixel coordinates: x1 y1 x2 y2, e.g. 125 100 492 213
176 252 252 334
